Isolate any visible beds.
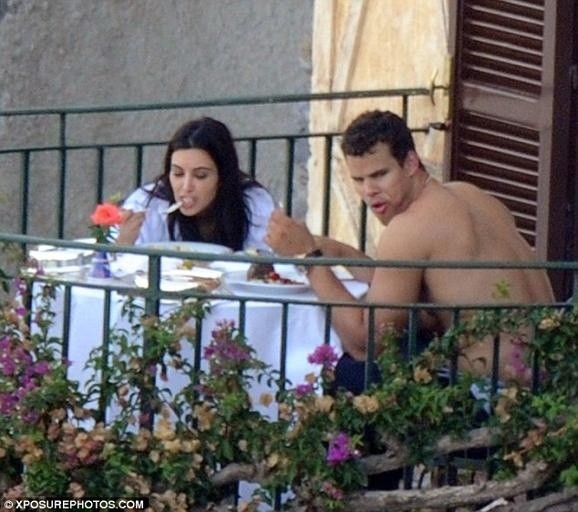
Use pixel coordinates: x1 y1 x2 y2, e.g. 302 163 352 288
20 239 369 437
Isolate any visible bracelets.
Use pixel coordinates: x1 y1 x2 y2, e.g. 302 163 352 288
294 249 323 277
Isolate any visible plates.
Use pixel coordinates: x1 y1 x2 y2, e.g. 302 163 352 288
136 240 234 271
239 282 307 295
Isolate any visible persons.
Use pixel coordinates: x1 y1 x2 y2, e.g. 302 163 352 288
101 116 278 278
263 109 558 491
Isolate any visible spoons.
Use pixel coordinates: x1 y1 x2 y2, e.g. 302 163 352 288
129 202 182 214
